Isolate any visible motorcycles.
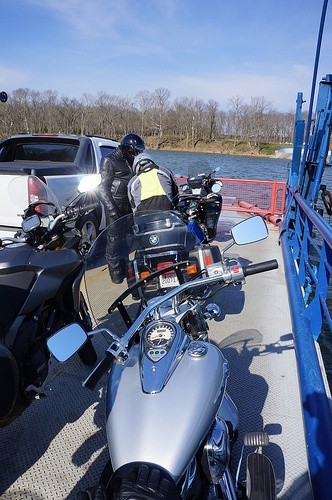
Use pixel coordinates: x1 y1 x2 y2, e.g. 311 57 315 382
124 166 224 297
45 207 278 500
0 173 111 428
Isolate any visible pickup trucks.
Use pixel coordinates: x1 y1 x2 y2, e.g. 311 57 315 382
0 133 122 246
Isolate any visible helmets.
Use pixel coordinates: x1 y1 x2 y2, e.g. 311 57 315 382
132 153 159 176
120 133 145 155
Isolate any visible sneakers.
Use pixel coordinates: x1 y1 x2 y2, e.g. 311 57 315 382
132 290 140 300
108 265 124 284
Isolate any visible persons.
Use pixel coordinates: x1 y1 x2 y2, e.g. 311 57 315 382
127 153 180 300
96 133 145 284
